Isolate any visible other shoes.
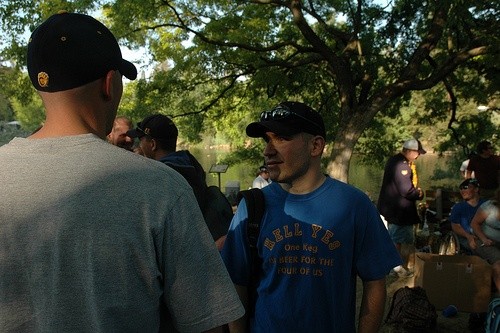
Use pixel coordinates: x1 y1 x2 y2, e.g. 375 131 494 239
394 266 415 277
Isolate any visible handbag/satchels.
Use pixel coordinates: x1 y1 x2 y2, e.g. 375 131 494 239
385 286 437 332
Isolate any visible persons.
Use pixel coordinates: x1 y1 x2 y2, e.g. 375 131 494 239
106 101 500 333
0 12 246 333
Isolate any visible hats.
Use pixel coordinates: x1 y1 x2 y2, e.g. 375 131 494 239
403 139 426 154
27 13 137 92
459 178 480 186
125 114 178 138
245 102 326 141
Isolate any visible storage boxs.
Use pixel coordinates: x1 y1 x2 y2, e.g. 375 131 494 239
415 253 492 313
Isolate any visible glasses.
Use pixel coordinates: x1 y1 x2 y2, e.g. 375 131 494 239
488 147 493 149
460 185 469 190
260 105 322 129
